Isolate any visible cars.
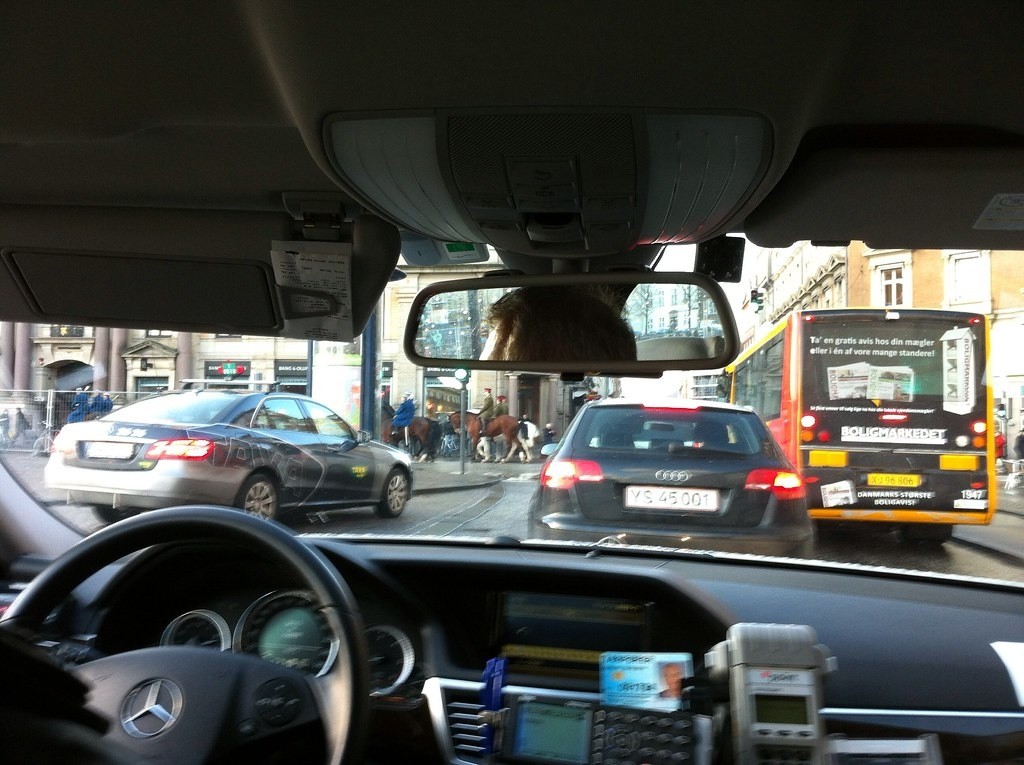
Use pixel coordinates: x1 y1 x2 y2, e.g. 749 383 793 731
528 396 815 561
43 362 414 525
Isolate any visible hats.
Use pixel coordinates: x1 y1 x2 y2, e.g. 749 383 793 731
103 392 110 396
484 388 492 392
497 396 506 400
402 392 413 400
426 401 434 404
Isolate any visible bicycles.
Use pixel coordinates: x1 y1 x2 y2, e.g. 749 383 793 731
34 421 61 456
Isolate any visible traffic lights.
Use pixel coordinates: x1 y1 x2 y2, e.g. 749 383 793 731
997 404 1006 417
750 290 764 304
454 368 470 382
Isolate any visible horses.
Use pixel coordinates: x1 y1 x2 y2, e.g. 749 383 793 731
380 399 443 465
44 395 112 438
447 405 541 466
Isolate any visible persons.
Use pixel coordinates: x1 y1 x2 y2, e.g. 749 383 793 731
518 415 530 421
0 409 10 433
994 420 1006 458
66 388 113 422
476 388 509 433
16 408 27 434
543 423 555 445
477 286 636 360
658 663 682 700
392 393 414 434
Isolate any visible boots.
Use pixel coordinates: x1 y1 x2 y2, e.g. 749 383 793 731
479 420 486 435
392 426 398 435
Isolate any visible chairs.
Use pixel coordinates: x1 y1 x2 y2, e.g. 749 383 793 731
597 420 730 450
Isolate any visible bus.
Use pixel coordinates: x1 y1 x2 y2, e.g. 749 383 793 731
714 305 997 551
